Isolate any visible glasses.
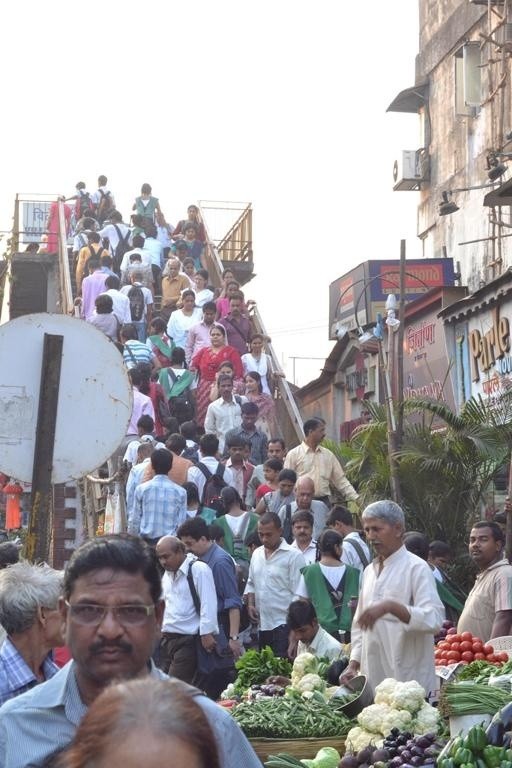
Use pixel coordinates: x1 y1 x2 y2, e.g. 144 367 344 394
63 600 160 627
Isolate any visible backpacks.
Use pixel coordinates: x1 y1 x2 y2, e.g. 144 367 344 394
153 336 173 368
194 462 228 517
84 246 103 278
113 224 134 273
181 445 200 462
98 188 114 218
127 285 145 322
75 191 93 219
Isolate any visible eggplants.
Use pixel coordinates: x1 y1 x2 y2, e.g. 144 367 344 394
382 727 441 768
483 703 512 747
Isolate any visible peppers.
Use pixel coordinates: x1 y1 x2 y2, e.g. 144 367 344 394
438 721 512 768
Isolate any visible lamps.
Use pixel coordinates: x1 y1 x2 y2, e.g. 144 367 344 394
332 268 431 344
439 151 512 217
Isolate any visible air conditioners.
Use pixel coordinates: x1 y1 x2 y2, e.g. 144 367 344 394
392 150 424 191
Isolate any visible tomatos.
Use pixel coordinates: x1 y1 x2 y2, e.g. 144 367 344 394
433 630 509 667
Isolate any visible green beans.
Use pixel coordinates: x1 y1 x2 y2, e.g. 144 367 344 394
229 695 354 739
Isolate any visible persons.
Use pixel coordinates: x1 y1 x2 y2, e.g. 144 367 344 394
2 478 71 706
51 679 223 767
243 419 448 706
0 535 262 767
56 173 283 691
454 522 512 644
26 196 71 252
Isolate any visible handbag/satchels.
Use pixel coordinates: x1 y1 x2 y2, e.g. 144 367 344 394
169 396 195 423
281 518 293 545
158 399 172 428
197 625 235 674
267 372 279 394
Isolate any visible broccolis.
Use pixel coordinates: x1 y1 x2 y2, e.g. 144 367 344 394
345 678 442 755
286 654 335 699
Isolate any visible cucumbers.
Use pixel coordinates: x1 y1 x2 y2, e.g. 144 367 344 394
264 752 314 767
